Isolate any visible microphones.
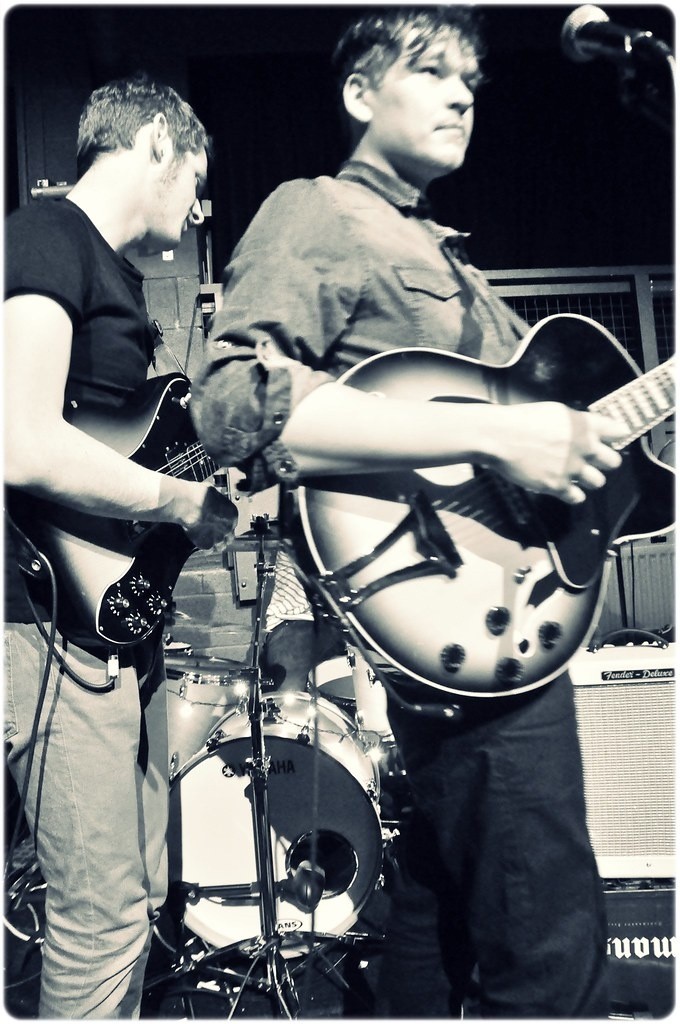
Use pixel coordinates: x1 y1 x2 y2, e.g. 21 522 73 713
291 859 326 909
561 5 671 63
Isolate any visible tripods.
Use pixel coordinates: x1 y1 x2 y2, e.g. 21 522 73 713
140 514 389 1019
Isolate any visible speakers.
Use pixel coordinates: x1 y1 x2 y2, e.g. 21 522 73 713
565 640 675 878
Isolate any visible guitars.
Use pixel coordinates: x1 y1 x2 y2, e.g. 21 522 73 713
3 373 265 656
298 312 676 700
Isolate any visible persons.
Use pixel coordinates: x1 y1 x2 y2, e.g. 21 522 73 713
4 80 239 1019
191 4 675 1020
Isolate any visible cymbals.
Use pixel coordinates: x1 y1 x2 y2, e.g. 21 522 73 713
161 610 233 630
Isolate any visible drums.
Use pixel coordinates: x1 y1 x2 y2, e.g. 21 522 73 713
143 689 383 964
307 650 403 721
163 654 257 778
